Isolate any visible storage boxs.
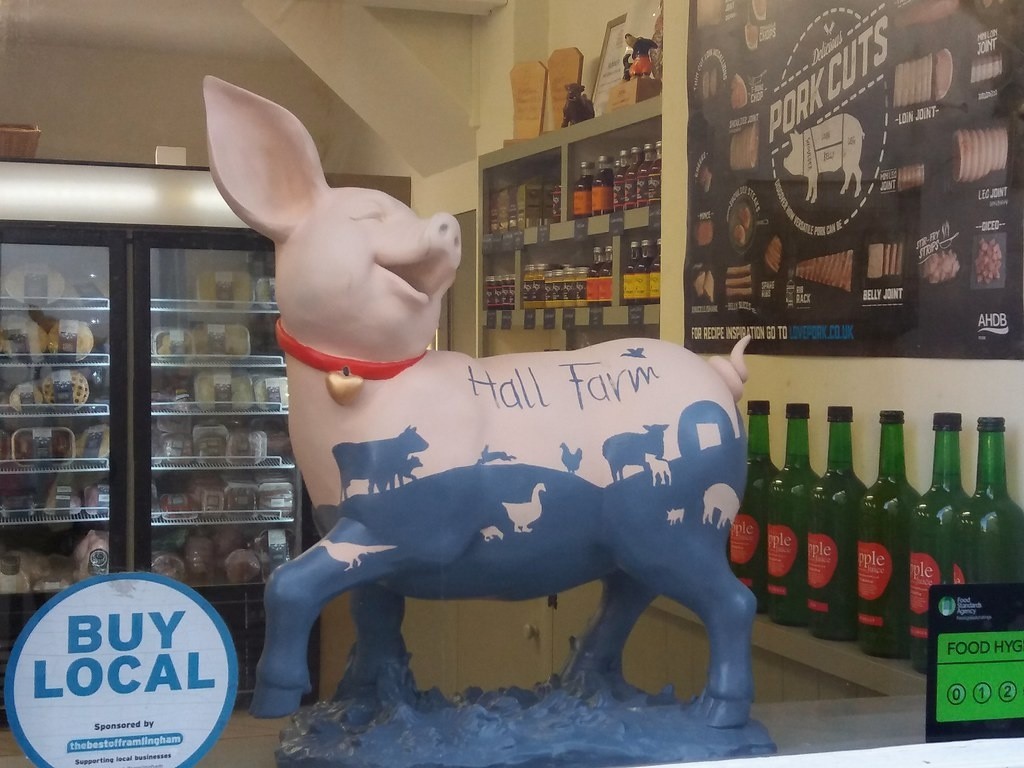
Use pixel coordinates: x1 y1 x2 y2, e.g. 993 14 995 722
488 175 561 234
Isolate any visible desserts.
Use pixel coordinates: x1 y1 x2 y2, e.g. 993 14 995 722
0 266 294 585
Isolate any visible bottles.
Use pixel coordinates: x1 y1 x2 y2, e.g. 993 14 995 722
650 238 660 304
623 241 642 305
856 410 922 658
647 140 661 206
637 144 656 207
806 406 869 640
615 160 621 173
768 404 822 625
613 150 629 211
573 161 593 217
624 147 643 210
592 155 613 215
954 417 1023 583
912 413 971 674
600 246 613 306
586 247 605 307
635 240 654 304
729 400 780 614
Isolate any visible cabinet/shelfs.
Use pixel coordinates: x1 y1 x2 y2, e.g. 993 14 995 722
476 95 663 359
0 156 321 726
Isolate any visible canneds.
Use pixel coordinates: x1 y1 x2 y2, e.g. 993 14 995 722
486 264 590 304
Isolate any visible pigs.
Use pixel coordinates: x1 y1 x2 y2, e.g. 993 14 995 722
203 75 759 738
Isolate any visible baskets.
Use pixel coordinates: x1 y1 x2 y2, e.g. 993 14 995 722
0 123 42 159
